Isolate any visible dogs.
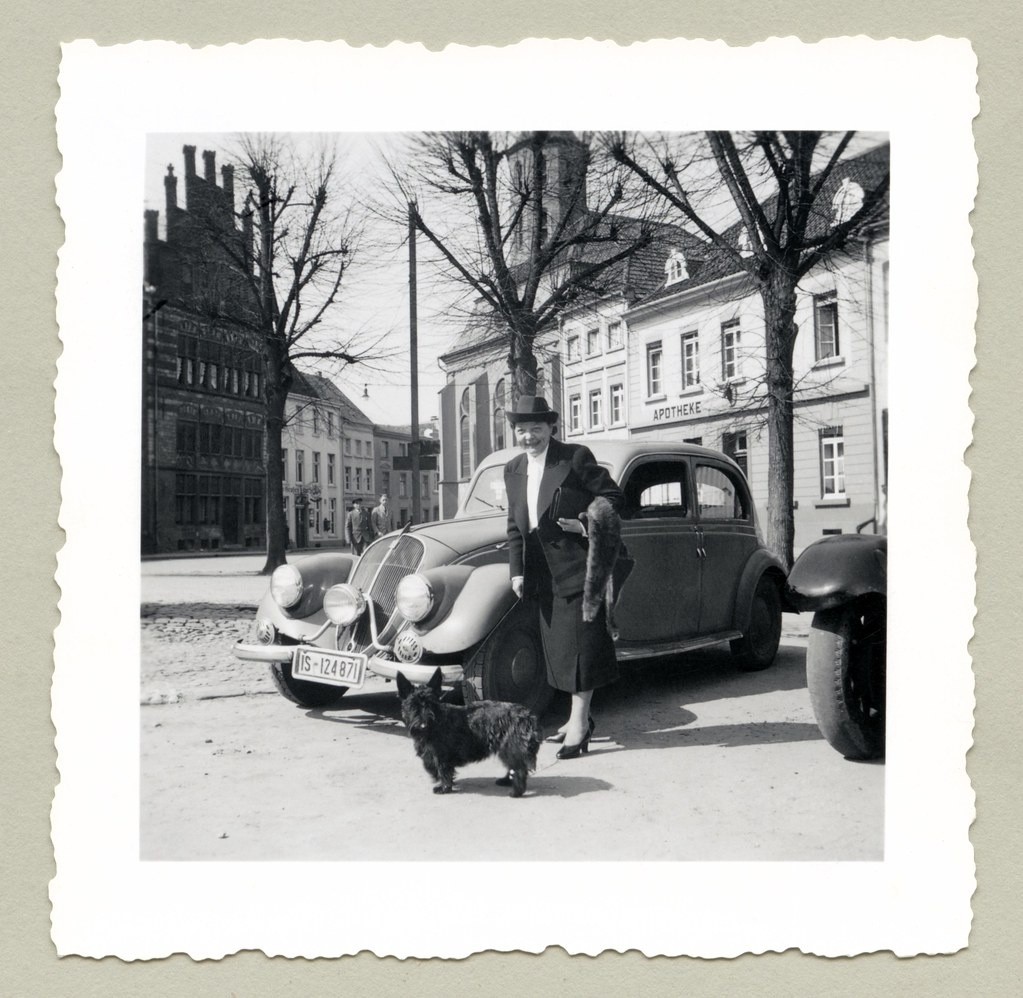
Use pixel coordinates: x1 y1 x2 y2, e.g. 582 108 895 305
394 669 541 798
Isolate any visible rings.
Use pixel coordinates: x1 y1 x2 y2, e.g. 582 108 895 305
563 528 567 531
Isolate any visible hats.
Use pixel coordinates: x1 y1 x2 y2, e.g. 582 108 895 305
505 395 559 424
351 496 363 504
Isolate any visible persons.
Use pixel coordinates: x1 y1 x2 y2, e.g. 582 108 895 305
397 522 401 529
502 393 624 761
344 498 374 556
372 492 395 539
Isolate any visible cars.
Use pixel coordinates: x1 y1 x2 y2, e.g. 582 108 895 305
231 442 788 743
783 533 886 764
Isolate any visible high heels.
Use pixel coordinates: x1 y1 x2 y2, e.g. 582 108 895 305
556 724 593 759
546 716 595 744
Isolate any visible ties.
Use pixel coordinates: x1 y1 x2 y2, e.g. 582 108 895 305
358 510 362 518
384 506 387 515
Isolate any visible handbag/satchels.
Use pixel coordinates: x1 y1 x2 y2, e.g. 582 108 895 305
548 486 591 520
